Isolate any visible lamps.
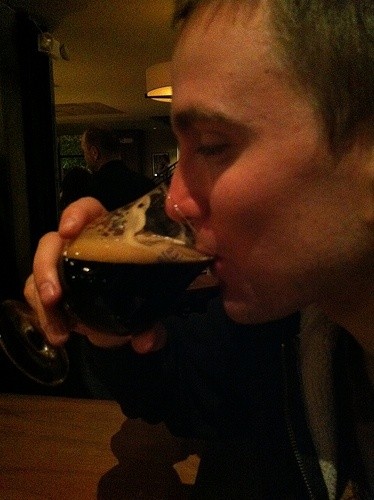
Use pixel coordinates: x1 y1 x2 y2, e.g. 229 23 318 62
144 62 173 103
38 31 70 61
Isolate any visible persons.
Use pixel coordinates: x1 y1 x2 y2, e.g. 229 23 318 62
24 0 374 500
58 124 180 237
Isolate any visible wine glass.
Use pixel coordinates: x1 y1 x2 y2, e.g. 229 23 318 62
0 182 214 388
